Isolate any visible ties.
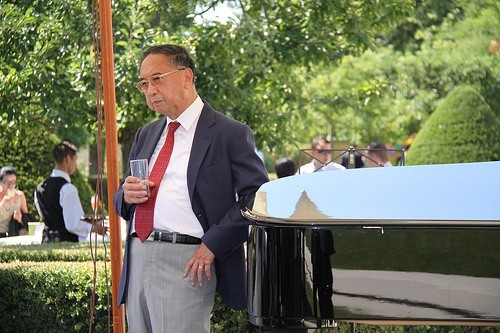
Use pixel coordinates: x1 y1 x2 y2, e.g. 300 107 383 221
133 121 182 244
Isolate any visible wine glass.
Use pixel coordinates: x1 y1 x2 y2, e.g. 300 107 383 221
7 189 18 209
90 194 100 219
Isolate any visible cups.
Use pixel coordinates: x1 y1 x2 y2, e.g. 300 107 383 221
129 159 150 196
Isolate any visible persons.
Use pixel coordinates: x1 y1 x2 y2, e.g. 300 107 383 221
34 141 111 243
113 44 269 333
0 166 29 238
296 136 346 176
361 142 392 168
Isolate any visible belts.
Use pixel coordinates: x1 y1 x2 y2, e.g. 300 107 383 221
130 230 205 245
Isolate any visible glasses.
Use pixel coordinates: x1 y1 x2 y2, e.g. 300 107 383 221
314 149 332 154
135 67 186 93
1 180 16 185
